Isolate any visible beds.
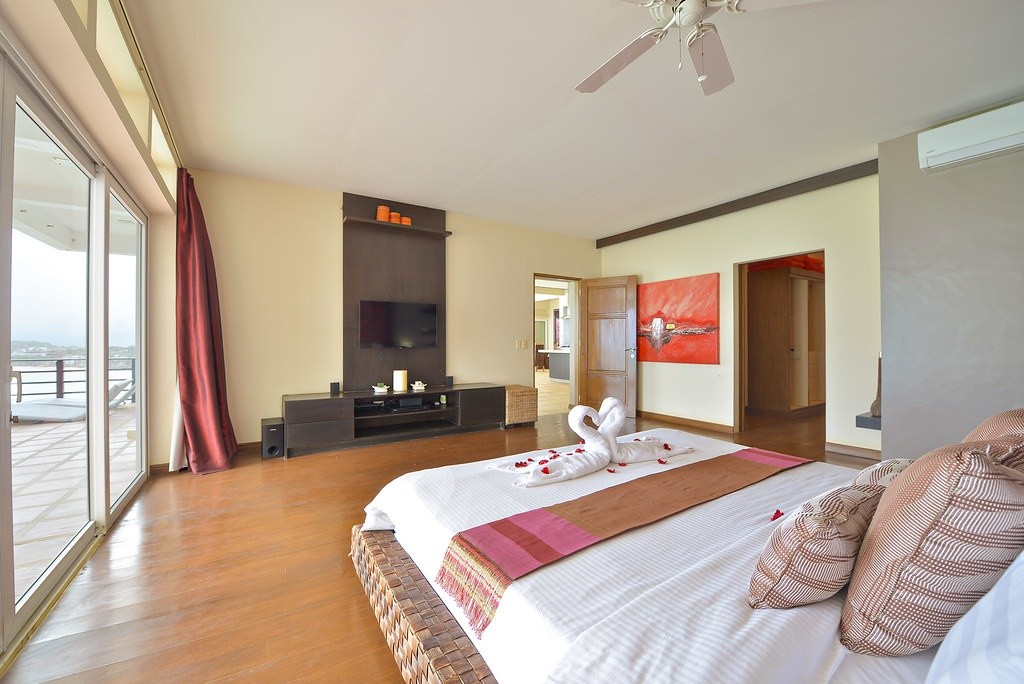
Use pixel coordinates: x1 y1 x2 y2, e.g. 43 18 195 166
360 427 1023 684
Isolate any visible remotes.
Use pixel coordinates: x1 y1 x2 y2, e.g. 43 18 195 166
435 401 439 405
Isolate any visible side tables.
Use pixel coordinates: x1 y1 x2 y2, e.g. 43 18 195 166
503 383 540 431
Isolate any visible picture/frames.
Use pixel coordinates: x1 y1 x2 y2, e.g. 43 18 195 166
638 270 720 365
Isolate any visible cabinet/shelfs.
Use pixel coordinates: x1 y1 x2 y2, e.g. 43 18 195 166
748 267 826 412
282 381 506 459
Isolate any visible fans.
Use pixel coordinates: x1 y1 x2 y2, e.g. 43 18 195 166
574 0 833 97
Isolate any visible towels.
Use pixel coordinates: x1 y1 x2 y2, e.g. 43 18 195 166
484 405 612 489
598 396 693 464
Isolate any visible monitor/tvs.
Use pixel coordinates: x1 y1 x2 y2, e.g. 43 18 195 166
358 299 438 349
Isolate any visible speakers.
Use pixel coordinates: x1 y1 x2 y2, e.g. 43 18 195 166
330 382 339 395
260 417 284 459
445 375 453 388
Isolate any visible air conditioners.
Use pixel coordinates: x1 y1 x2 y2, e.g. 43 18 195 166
917 100 1023 175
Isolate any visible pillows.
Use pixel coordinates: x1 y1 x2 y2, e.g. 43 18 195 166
747 406 1023 659
